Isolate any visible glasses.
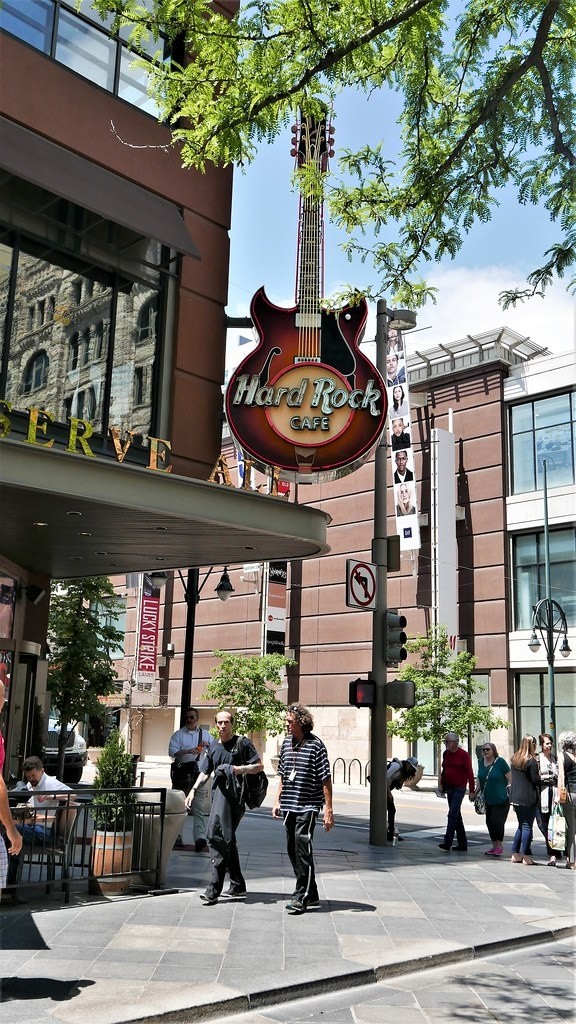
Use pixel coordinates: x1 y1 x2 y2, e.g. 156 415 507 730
442 740 457 743
288 706 304 716
185 715 198 721
482 748 493 751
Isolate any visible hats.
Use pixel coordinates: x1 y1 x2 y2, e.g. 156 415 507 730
407 757 418 770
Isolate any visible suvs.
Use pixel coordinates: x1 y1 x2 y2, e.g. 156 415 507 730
45 705 90 783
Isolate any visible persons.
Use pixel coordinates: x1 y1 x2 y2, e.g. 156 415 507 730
390 386 408 419
0 680 23 890
510 734 545 865
366 756 419 841
471 742 510 856
536 731 576 870
386 354 406 387
397 484 415 517
387 327 404 355
439 733 475 852
185 710 263 903
272 702 335 912
392 418 410 451
394 451 413 483
169 708 214 853
4 756 77 896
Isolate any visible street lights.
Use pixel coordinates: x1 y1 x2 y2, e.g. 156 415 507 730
528 458 572 754
147 564 239 730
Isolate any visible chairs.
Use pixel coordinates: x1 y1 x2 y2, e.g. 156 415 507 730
16 800 83 901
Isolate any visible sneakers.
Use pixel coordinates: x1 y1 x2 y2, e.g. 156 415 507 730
485 848 495 855
494 848 504 855
285 899 307 914
305 898 320 906
217 890 247 898
199 894 218 902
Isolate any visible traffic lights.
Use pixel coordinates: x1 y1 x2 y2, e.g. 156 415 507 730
346 678 381 708
381 608 410 667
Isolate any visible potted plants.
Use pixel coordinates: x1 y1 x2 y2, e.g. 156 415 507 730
90 724 141 893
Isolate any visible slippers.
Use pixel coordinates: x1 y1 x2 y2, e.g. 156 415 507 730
511 853 522 863
523 855 537 865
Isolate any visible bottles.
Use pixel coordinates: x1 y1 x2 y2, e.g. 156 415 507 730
392 828 399 847
196 742 203 752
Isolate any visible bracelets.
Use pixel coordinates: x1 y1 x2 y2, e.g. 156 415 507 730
53 794 56 800
191 787 197 790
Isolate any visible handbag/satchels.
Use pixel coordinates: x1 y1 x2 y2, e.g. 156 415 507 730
559 787 568 804
170 761 199 774
474 793 487 815
243 764 268 810
548 814 564 851
366 764 400 782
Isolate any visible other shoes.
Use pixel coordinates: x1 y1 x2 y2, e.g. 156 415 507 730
439 843 451 851
387 833 404 841
194 838 207 853
172 834 185 850
548 861 555 866
566 863 571 869
452 843 467 851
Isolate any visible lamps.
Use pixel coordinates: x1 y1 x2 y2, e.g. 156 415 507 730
18 575 46 606
